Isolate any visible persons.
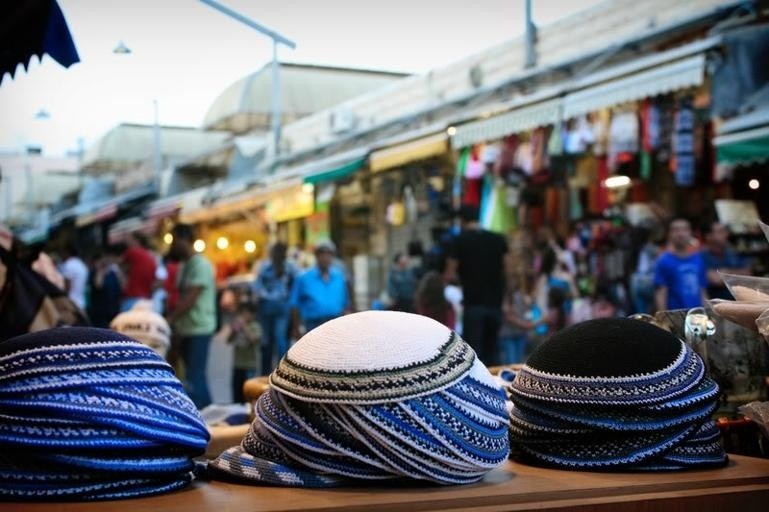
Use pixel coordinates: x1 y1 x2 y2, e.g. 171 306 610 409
0 195 769 411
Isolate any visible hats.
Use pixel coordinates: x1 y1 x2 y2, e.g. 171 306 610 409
209 311 510 488
1 324 211 502
509 315 731 471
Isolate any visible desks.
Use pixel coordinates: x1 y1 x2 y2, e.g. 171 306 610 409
0 421 768 512
